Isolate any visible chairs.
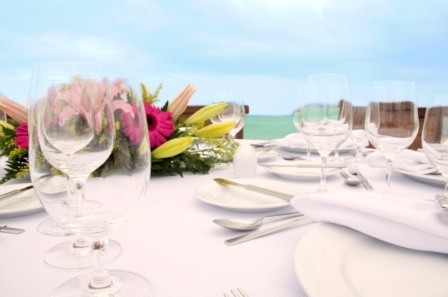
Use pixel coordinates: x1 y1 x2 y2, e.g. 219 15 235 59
338 99 448 150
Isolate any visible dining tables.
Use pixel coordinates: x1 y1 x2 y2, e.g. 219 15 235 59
0 138 448 297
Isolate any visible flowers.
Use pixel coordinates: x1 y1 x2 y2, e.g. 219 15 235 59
0 72 240 186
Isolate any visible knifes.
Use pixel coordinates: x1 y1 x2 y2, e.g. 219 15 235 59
0 185 34 199
214 177 293 201
223 216 313 245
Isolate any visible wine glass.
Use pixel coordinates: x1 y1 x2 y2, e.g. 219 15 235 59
291 72 353 199
210 98 246 146
341 85 380 166
365 80 419 201
26 74 153 297
421 104 448 208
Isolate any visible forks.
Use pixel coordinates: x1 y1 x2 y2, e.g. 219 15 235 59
221 285 254 297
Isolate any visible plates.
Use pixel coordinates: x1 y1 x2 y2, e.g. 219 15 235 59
293 222 448 297
267 160 347 181
393 157 448 187
197 177 297 212
274 132 359 152
0 182 46 218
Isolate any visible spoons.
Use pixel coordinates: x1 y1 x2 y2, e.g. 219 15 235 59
212 211 305 232
